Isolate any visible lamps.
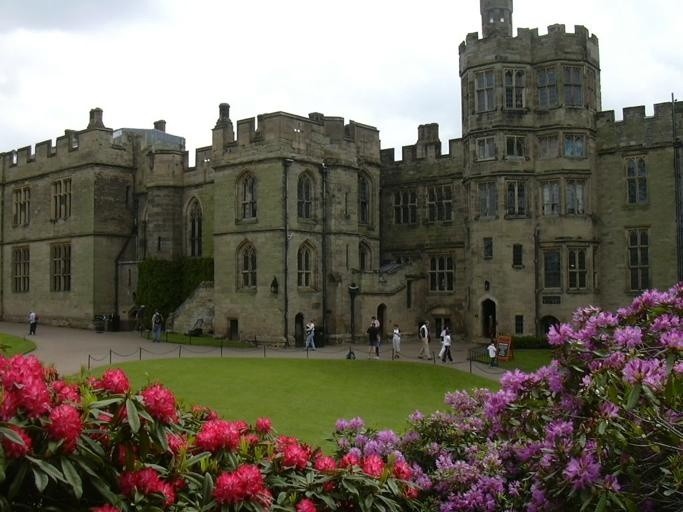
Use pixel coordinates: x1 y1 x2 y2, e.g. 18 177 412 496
485 281 489 290
271 276 278 293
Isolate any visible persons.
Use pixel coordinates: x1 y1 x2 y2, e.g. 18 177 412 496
391 323 401 359
366 316 382 360
303 321 317 351
488 314 495 339
417 320 454 364
151 308 164 343
486 340 499 369
26 312 37 336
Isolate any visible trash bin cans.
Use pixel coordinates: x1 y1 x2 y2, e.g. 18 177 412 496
94 312 119 333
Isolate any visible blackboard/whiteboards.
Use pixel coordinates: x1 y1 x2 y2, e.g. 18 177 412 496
495 336 510 361
